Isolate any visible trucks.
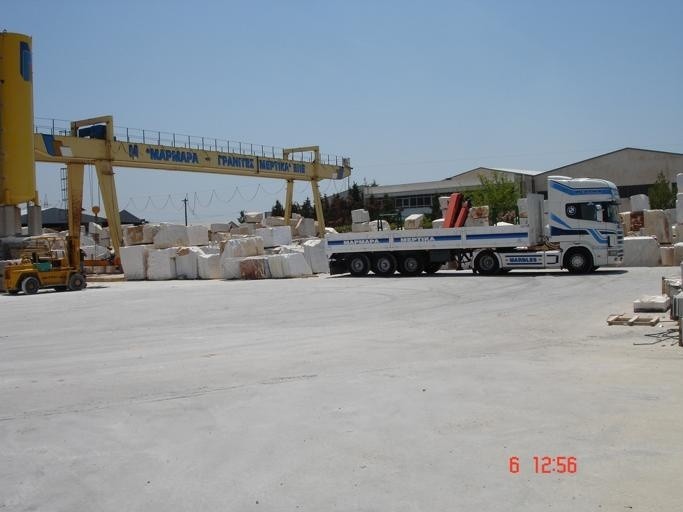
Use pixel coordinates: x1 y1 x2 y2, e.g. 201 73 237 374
322 176 625 275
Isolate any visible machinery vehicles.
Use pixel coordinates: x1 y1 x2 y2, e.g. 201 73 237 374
0 236 87 295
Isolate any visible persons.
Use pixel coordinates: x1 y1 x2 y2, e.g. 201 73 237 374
78 248 87 276
